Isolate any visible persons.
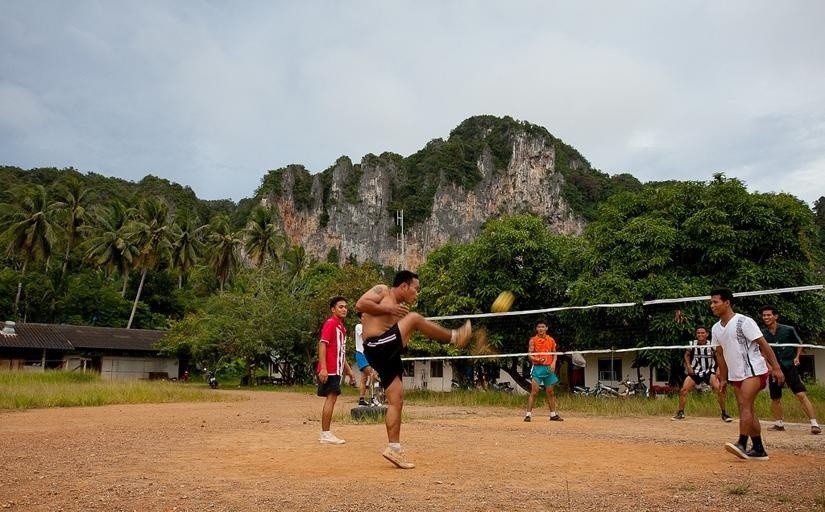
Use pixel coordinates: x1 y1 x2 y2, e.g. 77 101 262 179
314 296 356 445
669 324 733 423
709 286 785 461
354 270 472 469
522 318 564 422
354 310 380 407
756 305 821 434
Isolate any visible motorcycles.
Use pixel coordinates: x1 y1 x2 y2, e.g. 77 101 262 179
574 372 650 398
488 376 515 394
182 366 190 381
200 367 219 388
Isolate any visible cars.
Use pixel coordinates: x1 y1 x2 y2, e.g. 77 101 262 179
253 375 286 385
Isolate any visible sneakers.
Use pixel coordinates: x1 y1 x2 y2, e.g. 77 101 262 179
457 319 472 348
722 415 733 422
747 448 768 460
524 416 530 421
550 415 563 421
671 411 685 420
811 426 821 433
359 400 371 405
382 447 415 469
767 424 784 430
319 430 345 444
725 441 747 459
